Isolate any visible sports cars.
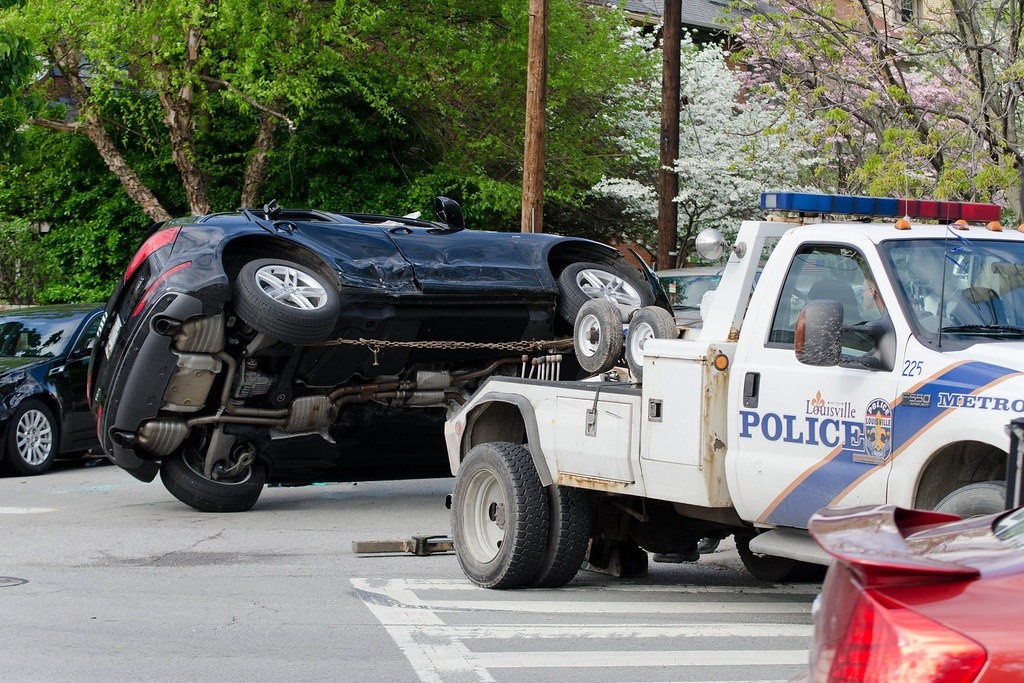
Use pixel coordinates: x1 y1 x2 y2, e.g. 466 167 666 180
806 504 1024 682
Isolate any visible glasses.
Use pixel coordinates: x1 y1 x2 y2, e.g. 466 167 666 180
859 288 878 295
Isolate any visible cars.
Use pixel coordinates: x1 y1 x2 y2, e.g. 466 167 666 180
84 206 678 513
647 265 877 348
0 299 109 477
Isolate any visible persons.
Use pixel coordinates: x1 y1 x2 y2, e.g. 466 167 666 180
858 273 889 322
655 538 724 564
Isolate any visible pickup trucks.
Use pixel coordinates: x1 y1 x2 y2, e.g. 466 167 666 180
443 191 1024 590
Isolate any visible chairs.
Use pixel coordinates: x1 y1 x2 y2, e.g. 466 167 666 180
950 287 1007 325
788 279 864 330
